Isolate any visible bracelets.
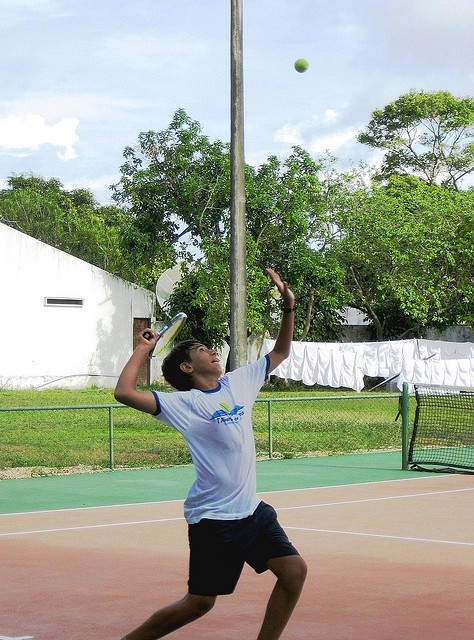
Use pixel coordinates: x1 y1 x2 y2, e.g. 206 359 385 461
279 305 297 313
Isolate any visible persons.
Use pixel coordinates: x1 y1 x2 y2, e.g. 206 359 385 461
115 268 308 640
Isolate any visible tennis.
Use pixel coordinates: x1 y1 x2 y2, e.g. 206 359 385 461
295 58 310 72
